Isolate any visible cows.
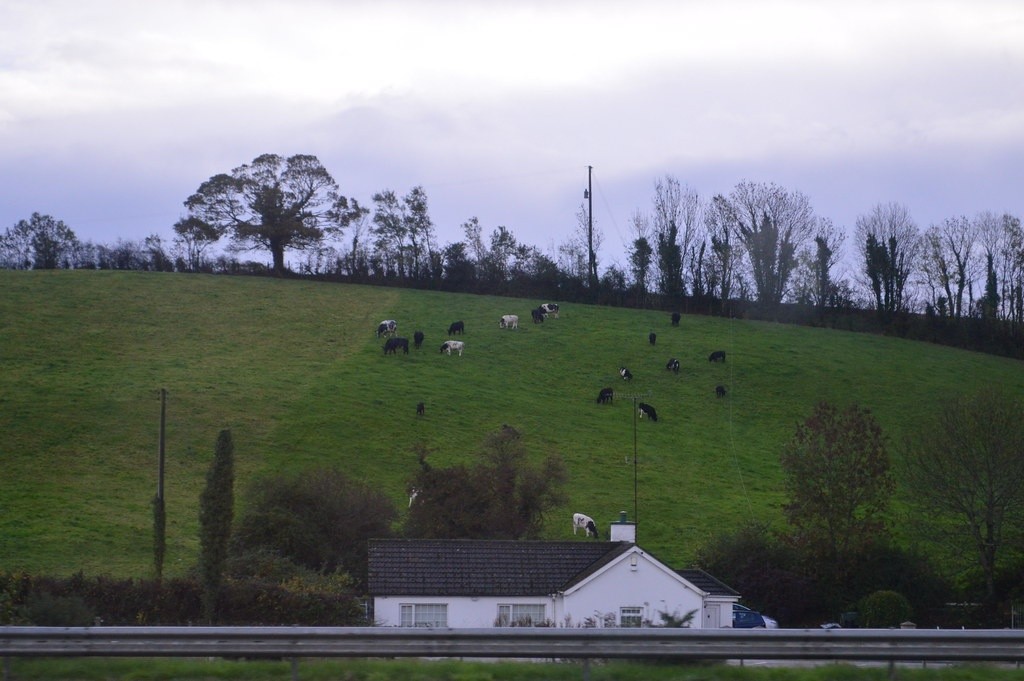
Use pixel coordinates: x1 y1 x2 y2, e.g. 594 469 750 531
648 333 656 344
530 302 560 324
638 401 658 423
416 401 425 414
666 357 680 373
596 387 614 403
671 311 682 329
617 366 634 382
708 350 726 363
570 513 602 539
375 318 467 358
715 385 727 397
498 315 518 330
406 486 423 512
